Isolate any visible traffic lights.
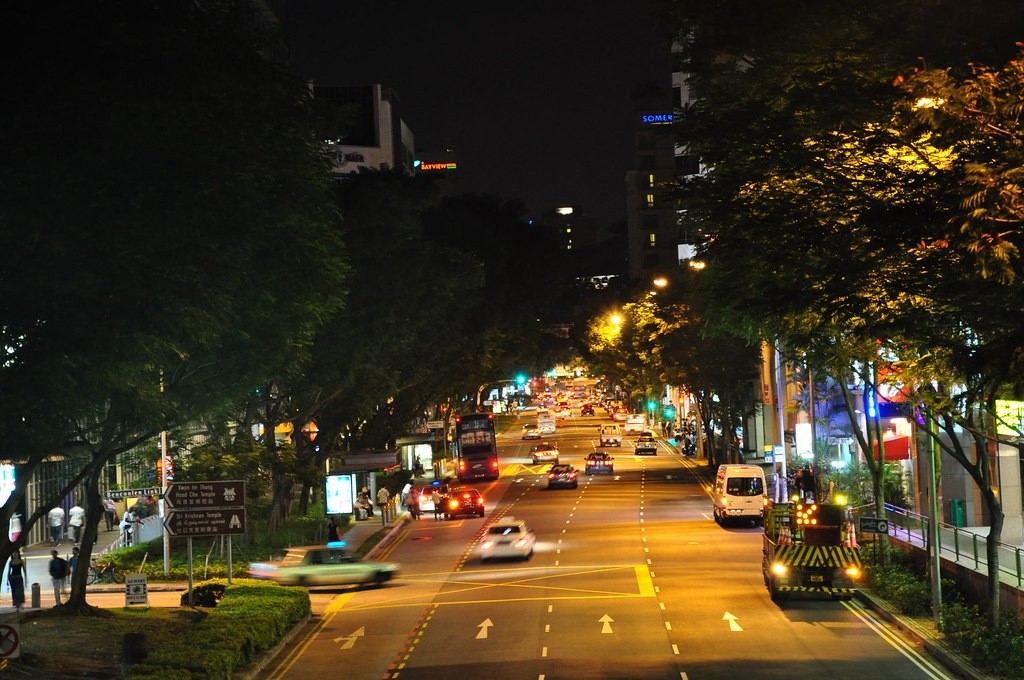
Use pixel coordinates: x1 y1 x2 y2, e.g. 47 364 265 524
647 401 656 411
518 376 526 384
662 408 674 419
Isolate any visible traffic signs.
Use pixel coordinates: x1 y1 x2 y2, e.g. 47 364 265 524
163 509 247 537
163 479 246 508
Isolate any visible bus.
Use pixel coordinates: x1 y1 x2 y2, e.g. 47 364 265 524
455 412 499 484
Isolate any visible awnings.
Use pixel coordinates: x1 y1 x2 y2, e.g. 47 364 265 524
861 434 909 461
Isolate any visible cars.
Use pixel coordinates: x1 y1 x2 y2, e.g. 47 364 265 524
479 516 536 565
520 424 542 440
634 436 659 456
245 545 401 589
418 482 450 513
532 375 647 435
546 464 579 490
530 444 559 465
444 486 485 520
584 452 614 474
692 417 744 450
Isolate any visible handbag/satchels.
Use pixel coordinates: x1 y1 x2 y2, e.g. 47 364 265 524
114 510 120 526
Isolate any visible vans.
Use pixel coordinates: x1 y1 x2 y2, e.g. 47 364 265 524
712 464 769 527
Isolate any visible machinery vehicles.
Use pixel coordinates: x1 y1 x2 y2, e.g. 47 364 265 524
760 501 863 602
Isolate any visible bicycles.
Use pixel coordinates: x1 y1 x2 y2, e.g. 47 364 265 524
85 553 126 586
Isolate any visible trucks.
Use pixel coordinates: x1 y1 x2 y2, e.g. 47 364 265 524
598 423 623 447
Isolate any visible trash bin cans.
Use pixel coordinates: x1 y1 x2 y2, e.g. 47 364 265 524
32 583 40 608
949 498 964 528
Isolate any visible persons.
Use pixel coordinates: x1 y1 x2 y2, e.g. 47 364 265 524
68 547 80 588
377 485 390 516
93 494 105 545
6 550 27 612
10 512 24 555
326 517 342 559
400 480 420 520
46 505 65 546
412 454 425 480
354 489 375 520
49 550 70 607
119 495 156 546
103 497 116 531
674 417 697 445
432 487 443 521
68 500 86 545
661 419 672 438
